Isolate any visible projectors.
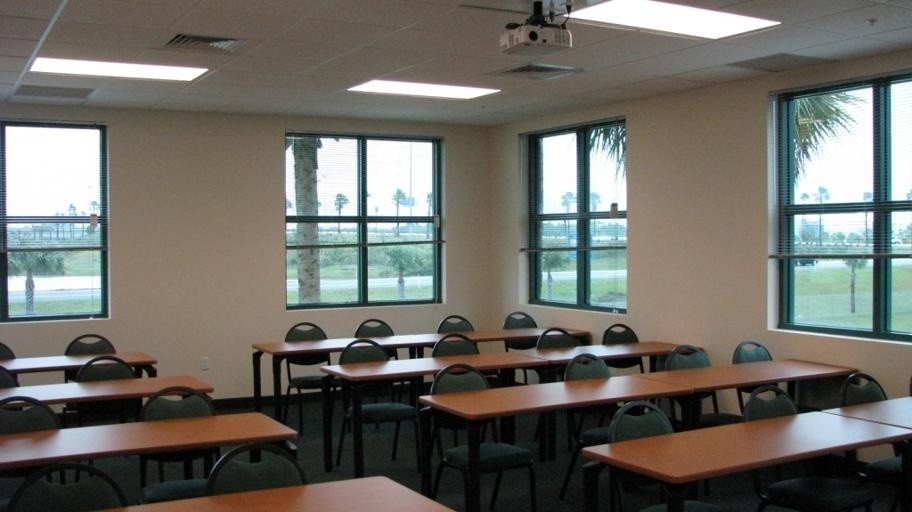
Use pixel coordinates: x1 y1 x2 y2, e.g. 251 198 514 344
500 23 572 55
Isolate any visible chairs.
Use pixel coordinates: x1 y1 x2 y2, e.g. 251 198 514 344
2 306 910 511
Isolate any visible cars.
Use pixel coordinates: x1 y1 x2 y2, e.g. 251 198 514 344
794 259 819 266
